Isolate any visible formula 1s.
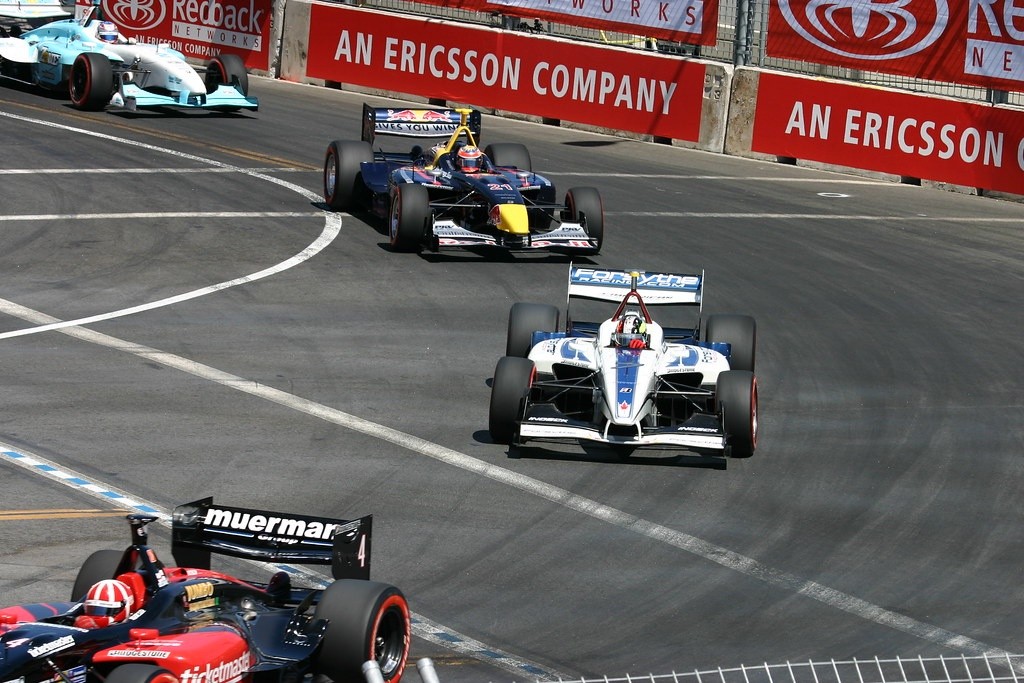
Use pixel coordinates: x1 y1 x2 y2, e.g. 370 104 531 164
323 102 604 256
0 1 260 115
0 497 414 683
489 261 760 458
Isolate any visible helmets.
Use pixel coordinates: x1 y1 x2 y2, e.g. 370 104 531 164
456 145 483 174
84 578 134 623
95 20 118 45
614 315 646 348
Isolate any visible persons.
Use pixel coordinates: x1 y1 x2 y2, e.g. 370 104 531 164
74 578 135 630
94 21 137 44
457 145 497 174
616 314 647 350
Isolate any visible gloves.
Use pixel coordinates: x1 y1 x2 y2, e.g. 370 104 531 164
72 616 99 631
629 339 645 350
